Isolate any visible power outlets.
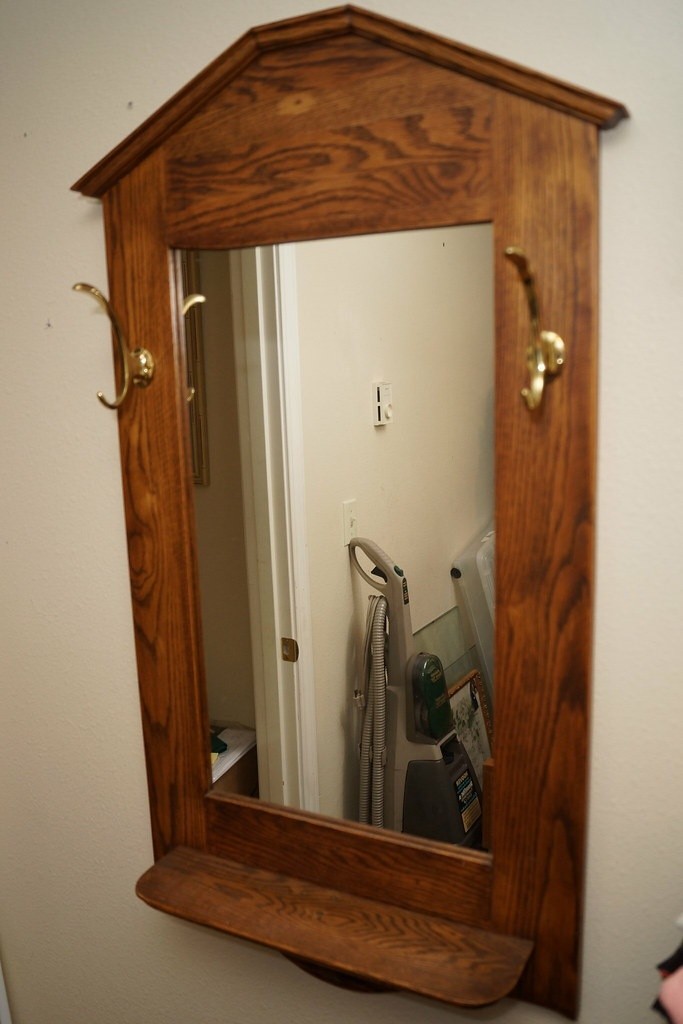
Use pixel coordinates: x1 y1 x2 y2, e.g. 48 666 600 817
342 498 358 546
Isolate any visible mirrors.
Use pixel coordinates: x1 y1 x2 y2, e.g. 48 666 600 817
168 221 496 866
